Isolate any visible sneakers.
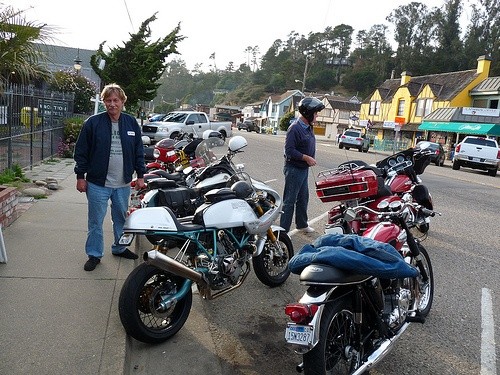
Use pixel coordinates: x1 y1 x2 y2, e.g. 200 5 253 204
296 226 315 232
285 233 291 240
84 256 100 270
111 248 138 259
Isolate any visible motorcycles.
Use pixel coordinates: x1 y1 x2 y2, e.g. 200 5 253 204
285 147 441 375
118 129 295 346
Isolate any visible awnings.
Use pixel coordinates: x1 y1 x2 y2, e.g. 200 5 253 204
418 122 500 136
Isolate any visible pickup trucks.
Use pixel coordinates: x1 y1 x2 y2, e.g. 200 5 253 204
139 111 233 146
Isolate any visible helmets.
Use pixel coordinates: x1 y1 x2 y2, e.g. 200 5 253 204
298 98 325 121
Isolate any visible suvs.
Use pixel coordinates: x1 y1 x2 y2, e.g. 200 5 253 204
452 136 500 177
338 129 370 153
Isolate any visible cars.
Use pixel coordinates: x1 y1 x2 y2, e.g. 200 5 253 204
236 120 261 133
413 141 446 166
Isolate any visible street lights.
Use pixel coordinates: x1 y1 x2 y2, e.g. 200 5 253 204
295 49 315 101
73 47 81 77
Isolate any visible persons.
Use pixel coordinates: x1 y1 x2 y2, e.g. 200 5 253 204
74 85 146 271
280 97 325 241
